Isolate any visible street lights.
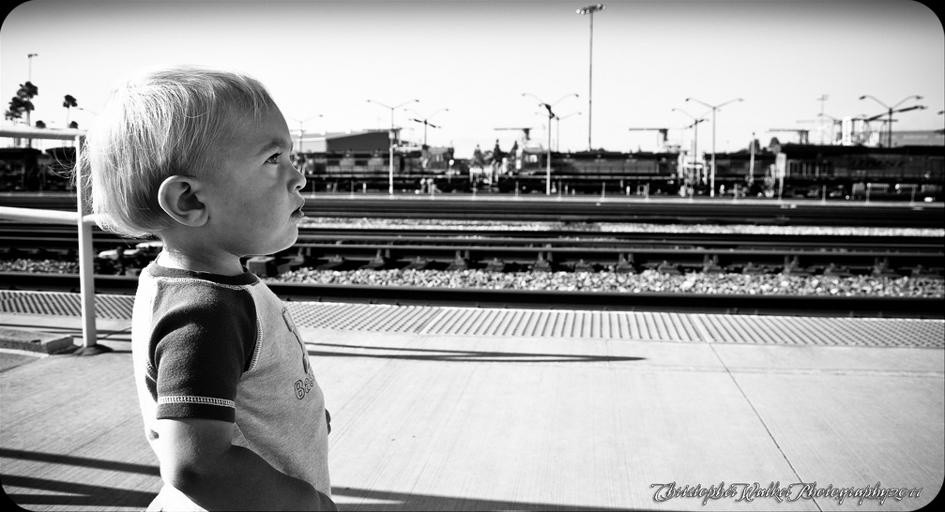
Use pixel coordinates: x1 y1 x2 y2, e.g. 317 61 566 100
521 92 582 194
402 106 448 148
793 94 922 147
685 97 743 198
366 97 419 194
671 108 721 163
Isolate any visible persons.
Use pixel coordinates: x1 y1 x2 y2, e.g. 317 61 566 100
77 69 339 512
